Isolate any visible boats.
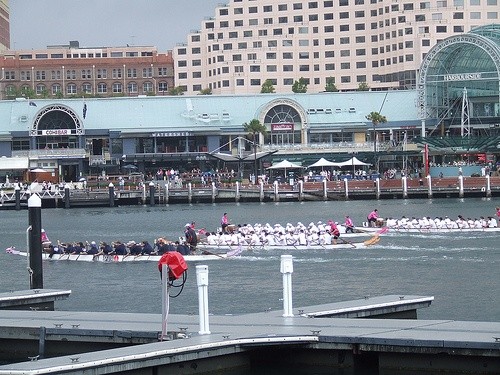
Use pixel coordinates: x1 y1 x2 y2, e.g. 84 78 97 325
6 246 241 264
340 221 500 233
155 234 385 252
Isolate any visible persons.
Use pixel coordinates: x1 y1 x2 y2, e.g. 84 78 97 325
198 227 207 235
183 221 196 233
428 159 500 177
400 167 415 181
367 208 383 228
135 166 236 191
205 219 347 246
494 207 500 228
41 228 49 253
220 212 231 232
381 215 498 229
48 237 194 258
344 216 354 233
249 167 397 187
184 226 198 252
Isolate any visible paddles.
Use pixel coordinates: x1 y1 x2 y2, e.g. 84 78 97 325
389 223 485 233
186 242 224 260
201 233 356 252
43 239 157 262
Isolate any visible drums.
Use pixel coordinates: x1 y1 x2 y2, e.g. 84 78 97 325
227 225 235 232
42 242 51 250
376 218 383 226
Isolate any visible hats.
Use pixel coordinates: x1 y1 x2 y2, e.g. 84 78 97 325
247 221 327 227
184 223 190 227
41 228 45 232
131 241 135 244
91 241 95 245
